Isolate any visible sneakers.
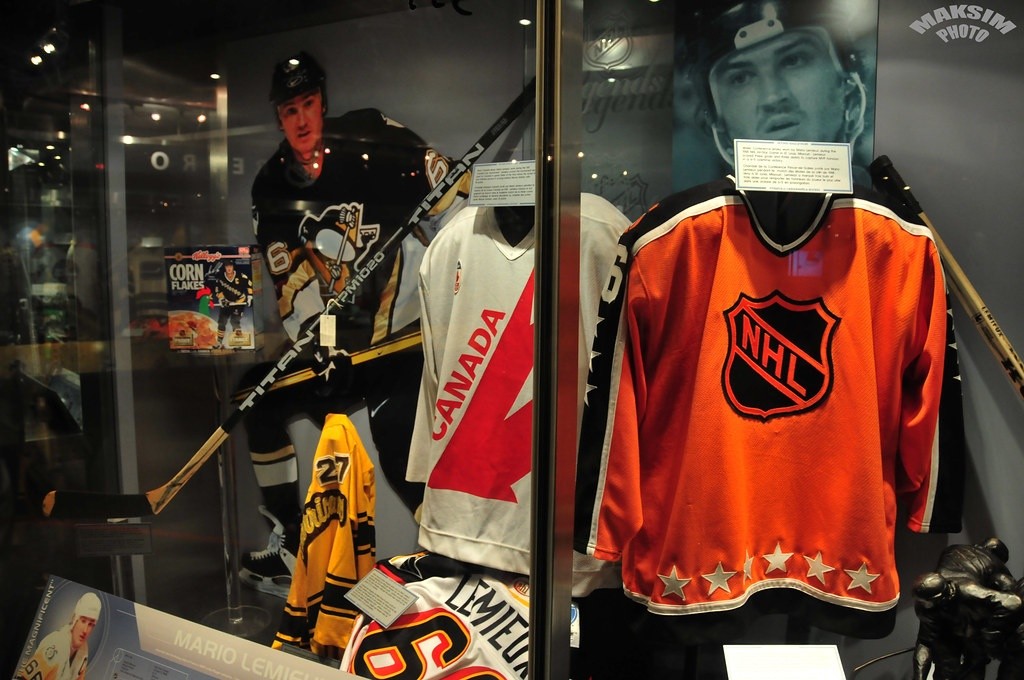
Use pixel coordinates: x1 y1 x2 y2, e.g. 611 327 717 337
212 338 222 349
238 504 293 599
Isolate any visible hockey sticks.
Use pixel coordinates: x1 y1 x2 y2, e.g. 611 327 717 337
866 154 1024 400
208 302 248 306
44 79 547 520
211 330 422 412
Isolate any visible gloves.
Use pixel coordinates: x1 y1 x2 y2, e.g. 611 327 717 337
295 319 356 384
217 292 228 308
246 294 253 307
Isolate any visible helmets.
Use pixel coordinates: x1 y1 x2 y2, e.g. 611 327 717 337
73 593 102 623
223 259 233 266
684 0 863 130
269 53 323 104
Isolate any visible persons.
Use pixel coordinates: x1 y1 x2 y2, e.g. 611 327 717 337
697 0 866 169
19 592 102 680
240 50 473 601
212 259 253 349
20 307 83 441
